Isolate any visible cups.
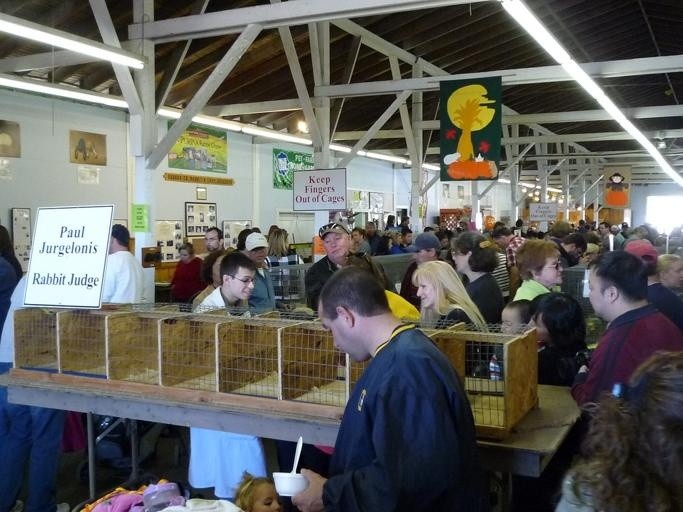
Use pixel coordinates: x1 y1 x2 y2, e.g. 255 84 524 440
142 482 182 512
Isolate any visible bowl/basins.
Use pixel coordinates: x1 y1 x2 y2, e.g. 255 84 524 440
271 472 308 496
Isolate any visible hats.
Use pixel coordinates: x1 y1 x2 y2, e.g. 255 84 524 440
246 232 270 252
404 232 441 252
319 223 348 239
623 239 659 265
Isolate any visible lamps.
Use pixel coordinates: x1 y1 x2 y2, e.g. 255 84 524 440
0 0 130 109
157 106 242 132
329 143 411 164
241 123 312 146
0 9 149 71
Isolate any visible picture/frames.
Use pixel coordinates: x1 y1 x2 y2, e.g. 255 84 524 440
1 120 21 158
220 217 252 249
69 130 107 164
184 201 217 236
9 205 31 273
196 186 208 200
153 217 186 264
111 218 128 233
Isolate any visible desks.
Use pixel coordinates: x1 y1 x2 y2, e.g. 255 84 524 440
1 352 584 512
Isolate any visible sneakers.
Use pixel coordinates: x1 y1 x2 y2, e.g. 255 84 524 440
7 499 24 512
55 502 71 512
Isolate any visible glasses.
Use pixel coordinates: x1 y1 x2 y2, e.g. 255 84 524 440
229 274 255 286
544 260 562 270
